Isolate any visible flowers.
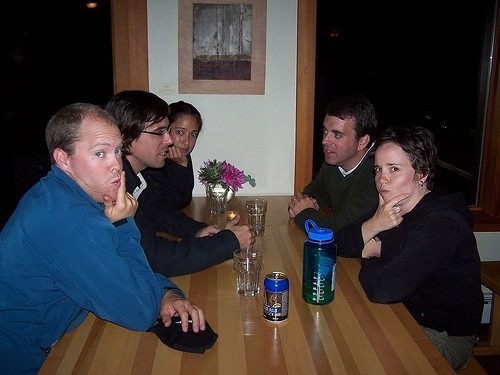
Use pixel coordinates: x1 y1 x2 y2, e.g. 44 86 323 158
197 158 248 192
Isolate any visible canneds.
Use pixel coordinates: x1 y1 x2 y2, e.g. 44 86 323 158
264 272 290 322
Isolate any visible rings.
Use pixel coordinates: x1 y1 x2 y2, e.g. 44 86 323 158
292 198 297 202
393 206 400 213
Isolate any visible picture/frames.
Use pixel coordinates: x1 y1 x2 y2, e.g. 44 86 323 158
177 1 267 95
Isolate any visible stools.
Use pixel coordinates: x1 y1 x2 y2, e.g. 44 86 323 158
455 356 489 375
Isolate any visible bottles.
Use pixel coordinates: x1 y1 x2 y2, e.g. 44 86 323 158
302 219 336 305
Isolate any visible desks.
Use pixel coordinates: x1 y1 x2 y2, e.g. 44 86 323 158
471 260 500 357
36 195 458 375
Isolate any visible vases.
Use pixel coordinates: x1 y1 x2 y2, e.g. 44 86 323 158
205 181 229 215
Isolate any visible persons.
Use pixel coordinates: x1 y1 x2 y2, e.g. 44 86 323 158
0 102 208 375
286 92 383 241
331 122 485 371
105 89 256 278
167 100 202 208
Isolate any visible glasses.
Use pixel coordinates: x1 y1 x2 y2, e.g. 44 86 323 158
139 126 172 137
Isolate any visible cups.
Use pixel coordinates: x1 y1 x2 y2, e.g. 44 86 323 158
247 198 267 233
233 249 263 296
